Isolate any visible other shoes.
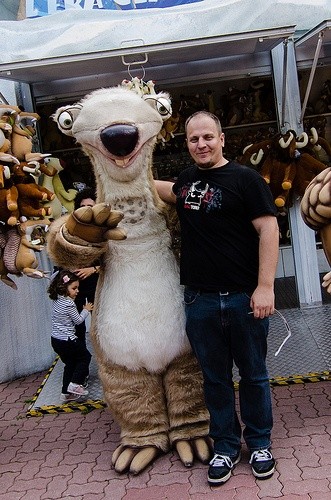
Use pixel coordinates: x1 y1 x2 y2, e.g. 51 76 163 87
67 382 89 396
61 393 80 401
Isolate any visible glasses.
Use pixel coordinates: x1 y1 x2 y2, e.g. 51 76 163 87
249 308 292 357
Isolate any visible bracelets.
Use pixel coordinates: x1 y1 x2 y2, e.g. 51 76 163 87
94 267 97 272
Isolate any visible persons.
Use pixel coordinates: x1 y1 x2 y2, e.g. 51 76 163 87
154 111 279 486
74 189 99 387
47 269 94 401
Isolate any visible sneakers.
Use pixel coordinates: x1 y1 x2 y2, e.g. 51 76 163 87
248 450 277 478
207 451 241 484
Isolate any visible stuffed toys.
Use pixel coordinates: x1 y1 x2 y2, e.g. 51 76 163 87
296 127 331 198
0 216 51 290
0 164 19 226
300 167 331 293
0 104 52 165
39 157 78 214
46 78 213 475
12 161 55 217
243 129 308 216
174 87 269 133
313 79 331 113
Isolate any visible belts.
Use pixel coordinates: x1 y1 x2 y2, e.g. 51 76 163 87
199 291 241 297
